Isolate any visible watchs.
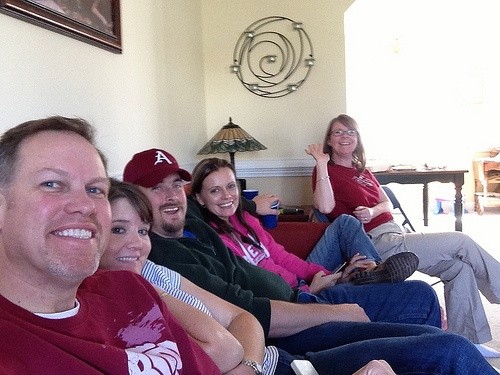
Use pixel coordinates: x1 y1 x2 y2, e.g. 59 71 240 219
241 361 263 375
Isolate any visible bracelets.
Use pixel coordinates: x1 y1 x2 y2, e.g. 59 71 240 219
316 176 329 181
161 292 168 296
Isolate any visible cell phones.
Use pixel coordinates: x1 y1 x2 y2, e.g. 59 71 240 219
332 260 346 275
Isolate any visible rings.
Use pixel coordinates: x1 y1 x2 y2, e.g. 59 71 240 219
365 218 367 220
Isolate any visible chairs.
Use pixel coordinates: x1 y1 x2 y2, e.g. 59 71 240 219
309 187 444 286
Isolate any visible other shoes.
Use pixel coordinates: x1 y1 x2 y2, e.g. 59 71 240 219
349 252 419 284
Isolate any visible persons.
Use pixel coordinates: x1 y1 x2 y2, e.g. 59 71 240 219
188 158 418 284
0 116 395 375
122 148 442 340
304 114 500 357
99 179 500 374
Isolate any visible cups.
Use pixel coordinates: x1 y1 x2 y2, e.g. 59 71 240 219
243 189 259 214
263 201 281 229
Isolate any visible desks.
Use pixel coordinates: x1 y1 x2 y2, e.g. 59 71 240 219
369 164 468 231
276 205 314 224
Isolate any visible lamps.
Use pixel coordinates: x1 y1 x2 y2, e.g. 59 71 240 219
198 117 268 186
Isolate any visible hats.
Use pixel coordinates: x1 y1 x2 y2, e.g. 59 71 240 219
123 149 192 188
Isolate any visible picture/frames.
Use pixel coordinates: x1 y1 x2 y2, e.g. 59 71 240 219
0 0 123 54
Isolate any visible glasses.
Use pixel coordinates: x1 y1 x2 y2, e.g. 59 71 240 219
331 129 356 136
